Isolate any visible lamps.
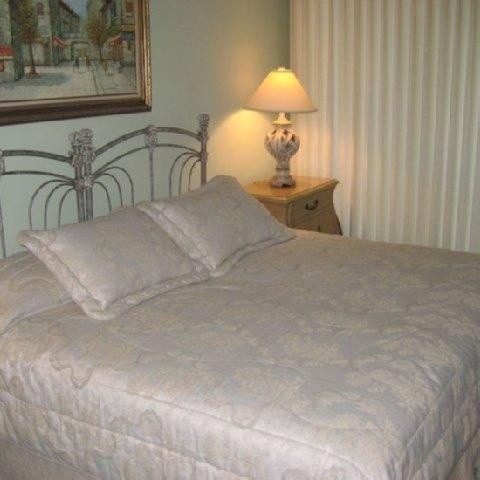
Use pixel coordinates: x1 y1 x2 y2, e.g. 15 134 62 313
244 61 321 192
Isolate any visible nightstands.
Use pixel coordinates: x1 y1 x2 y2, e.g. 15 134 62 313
243 173 345 238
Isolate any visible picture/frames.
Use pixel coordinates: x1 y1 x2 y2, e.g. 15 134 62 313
0 1 156 129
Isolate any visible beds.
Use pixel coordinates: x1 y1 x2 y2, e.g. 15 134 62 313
0 107 480 479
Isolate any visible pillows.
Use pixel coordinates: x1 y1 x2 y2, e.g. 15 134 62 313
14 205 209 324
142 171 298 282
